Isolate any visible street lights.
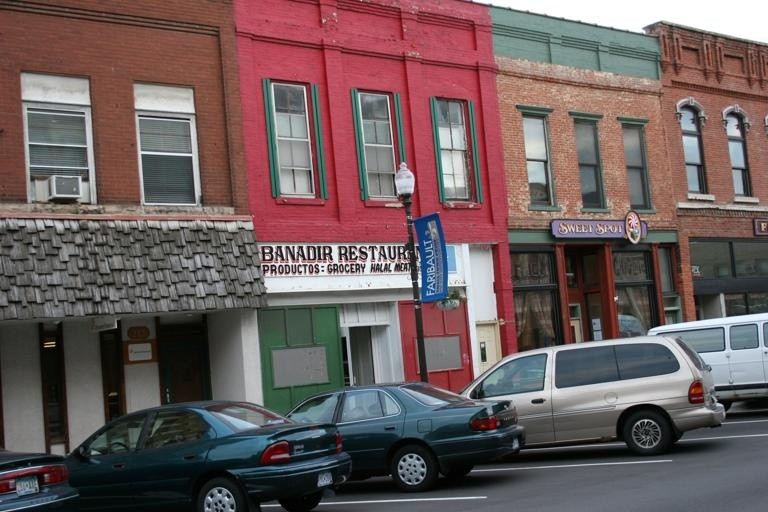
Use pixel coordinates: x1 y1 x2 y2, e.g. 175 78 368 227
390 160 428 382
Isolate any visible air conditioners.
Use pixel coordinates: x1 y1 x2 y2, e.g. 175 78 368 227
46 175 82 201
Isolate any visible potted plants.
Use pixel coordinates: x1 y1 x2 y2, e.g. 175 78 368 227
429 286 467 312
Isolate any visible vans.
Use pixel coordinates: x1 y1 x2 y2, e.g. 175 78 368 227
459 334 727 460
643 309 768 412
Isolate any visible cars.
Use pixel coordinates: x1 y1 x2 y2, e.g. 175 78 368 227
0 444 81 511
282 379 529 495
58 397 358 511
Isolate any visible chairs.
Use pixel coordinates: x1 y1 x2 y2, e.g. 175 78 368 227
349 404 382 419
